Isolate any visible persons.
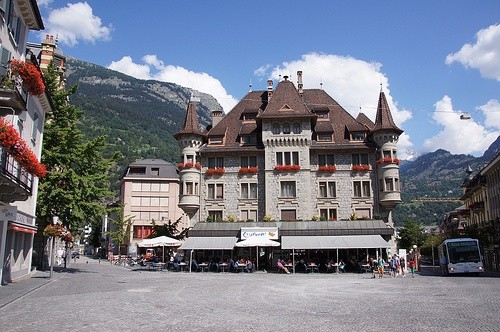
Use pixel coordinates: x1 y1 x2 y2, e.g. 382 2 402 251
440 253 446 276
409 256 415 278
388 254 406 278
277 258 319 274
128 254 132 262
222 255 252 273
138 250 159 266
181 257 221 273
348 255 387 279
326 258 345 273
162 253 179 272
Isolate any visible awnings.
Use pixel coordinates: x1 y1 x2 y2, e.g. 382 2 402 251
281 236 391 249
178 237 236 249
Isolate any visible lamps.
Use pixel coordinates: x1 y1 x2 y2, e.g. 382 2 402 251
460 112 471 119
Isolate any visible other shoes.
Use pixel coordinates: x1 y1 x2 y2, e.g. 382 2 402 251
287 272 290 274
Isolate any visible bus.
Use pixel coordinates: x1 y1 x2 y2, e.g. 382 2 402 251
437 238 484 276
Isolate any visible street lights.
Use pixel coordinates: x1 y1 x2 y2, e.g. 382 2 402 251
49 215 59 280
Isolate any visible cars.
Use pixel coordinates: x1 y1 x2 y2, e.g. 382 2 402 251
71 252 79 259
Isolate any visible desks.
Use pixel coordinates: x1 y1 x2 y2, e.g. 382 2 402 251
362 265 370 273
308 265 317 273
285 264 292 269
178 264 188 271
199 264 208 272
220 264 227 272
238 264 246 272
332 265 340 273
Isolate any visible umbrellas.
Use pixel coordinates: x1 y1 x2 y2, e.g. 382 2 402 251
236 237 280 270
138 236 182 269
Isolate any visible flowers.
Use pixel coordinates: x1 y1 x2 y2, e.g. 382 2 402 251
10 58 45 96
376 157 400 164
275 164 300 171
178 162 201 169
239 167 257 174
205 168 225 174
319 164 336 172
43 224 72 241
352 163 370 172
0 118 47 177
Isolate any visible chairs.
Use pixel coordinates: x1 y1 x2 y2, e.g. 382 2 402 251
150 257 365 273
